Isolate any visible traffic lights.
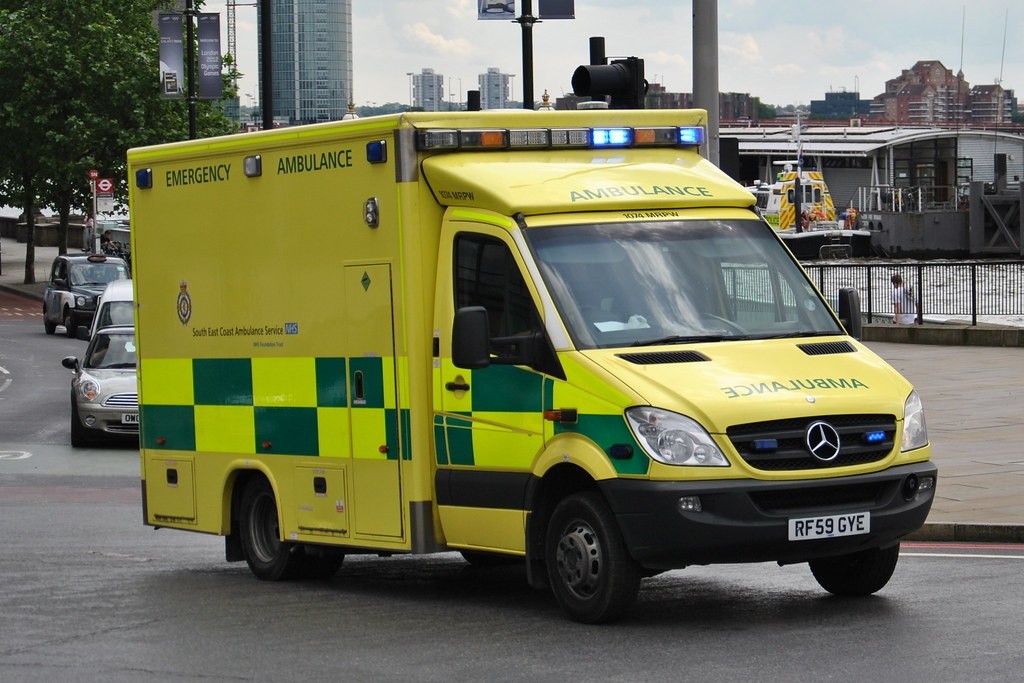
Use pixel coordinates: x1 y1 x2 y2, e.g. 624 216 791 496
572 58 644 109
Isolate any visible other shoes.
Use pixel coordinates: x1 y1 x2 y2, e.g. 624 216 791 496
82 248 85 251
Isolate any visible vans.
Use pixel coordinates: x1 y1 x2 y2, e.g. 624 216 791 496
76 278 136 342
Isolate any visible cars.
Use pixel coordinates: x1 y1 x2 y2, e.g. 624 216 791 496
61 323 142 448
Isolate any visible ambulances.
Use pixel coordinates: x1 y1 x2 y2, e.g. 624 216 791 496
126 106 938 626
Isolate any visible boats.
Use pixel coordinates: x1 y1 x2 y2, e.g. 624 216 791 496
741 169 872 261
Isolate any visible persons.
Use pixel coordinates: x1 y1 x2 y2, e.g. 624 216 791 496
81 213 93 252
101 229 118 249
890 275 918 324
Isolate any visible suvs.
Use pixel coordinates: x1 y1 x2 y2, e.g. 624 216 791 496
41 252 131 338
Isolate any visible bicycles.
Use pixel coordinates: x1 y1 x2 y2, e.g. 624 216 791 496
81 241 132 280
